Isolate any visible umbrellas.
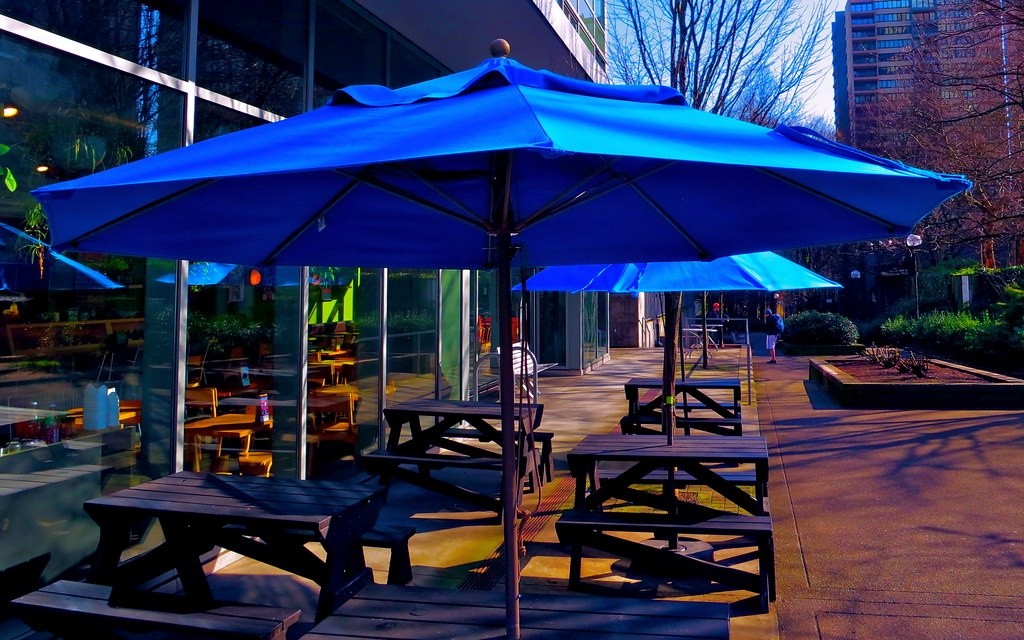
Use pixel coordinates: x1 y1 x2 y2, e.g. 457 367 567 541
31 36 972 637
513 251 844 447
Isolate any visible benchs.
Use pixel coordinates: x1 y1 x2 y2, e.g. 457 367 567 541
367 446 503 492
556 511 776 611
444 428 555 485
222 524 416 587
11 581 302 640
596 467 757 486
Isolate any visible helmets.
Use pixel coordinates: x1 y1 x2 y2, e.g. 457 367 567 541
713 303 720 307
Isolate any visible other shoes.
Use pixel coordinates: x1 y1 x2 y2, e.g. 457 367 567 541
767 360 777 364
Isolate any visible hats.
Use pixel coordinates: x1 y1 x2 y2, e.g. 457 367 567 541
764 309 772 314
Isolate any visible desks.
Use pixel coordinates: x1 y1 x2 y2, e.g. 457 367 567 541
299 584 730 640
383 398 544 507
184 413 275 438
566 434 770 514
625 377 742 418
82 470 387 628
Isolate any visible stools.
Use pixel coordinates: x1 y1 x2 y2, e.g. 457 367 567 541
64 322 362 478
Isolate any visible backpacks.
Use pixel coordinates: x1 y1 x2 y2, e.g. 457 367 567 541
771 314 785 334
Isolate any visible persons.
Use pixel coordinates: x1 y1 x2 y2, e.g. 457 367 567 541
764 308 784 364
